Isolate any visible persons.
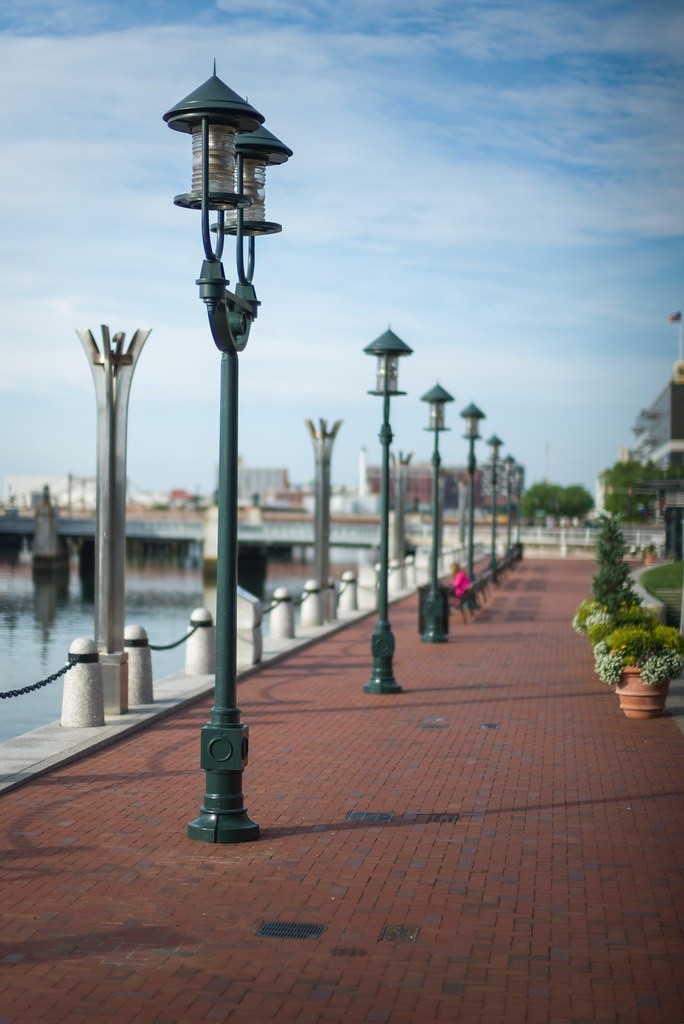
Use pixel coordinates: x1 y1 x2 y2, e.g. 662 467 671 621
645 544 656 566
449 562 470 614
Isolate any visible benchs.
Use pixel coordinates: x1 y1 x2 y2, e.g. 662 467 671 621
448 545 521 625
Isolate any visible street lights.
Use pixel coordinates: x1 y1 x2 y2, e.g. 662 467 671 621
361 321 415 696
161 54 293 845
419 380 450 643
457 402 485 581
486 435 526 589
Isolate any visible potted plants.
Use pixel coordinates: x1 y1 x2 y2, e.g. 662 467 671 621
572 514 684 721
